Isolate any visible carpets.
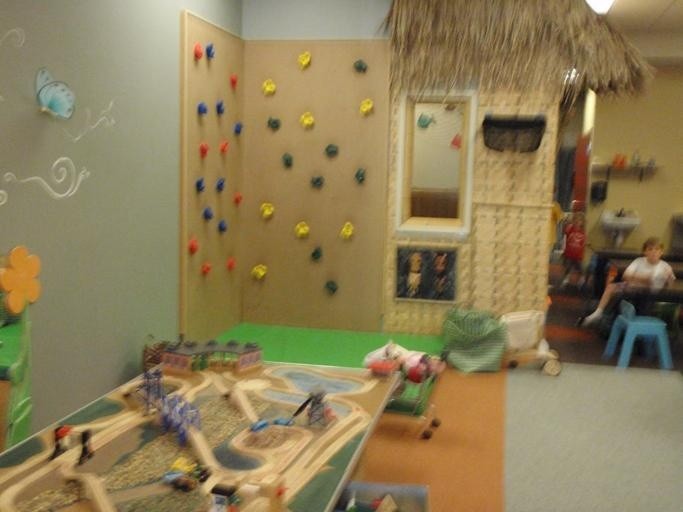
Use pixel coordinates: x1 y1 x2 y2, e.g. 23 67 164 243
505 362 683 512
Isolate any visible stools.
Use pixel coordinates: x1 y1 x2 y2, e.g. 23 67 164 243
600 314 674 370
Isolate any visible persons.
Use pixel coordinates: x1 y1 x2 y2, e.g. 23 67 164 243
561 212 587 289
576 237 676 328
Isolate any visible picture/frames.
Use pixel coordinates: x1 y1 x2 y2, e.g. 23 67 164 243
393 241 461 304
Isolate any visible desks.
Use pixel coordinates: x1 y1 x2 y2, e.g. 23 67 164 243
0 358 404 512
616 288 683 304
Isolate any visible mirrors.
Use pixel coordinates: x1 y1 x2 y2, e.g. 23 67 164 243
401 96 471 228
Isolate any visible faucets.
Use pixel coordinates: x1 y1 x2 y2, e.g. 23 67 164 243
616 209 626 217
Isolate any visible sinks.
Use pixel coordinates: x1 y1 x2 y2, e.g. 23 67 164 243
602 217 640 223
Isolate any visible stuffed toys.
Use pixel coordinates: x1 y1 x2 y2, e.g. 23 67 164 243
361 339 446 383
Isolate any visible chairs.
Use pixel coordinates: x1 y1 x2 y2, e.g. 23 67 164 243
592 212 683 300
